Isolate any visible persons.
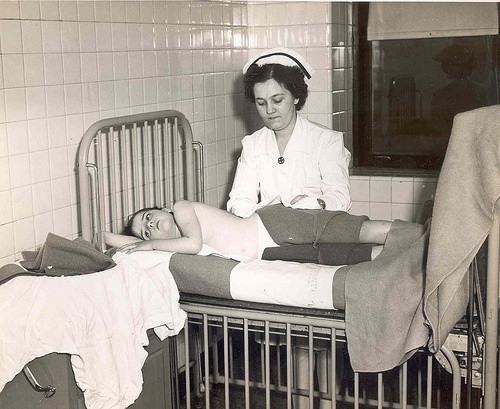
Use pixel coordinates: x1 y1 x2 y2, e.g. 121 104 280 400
225 46 355 409
113 199 398 265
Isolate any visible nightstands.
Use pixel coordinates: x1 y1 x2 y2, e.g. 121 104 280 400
0 251 172 409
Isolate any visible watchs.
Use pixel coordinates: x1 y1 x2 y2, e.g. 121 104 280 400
317 198 326 210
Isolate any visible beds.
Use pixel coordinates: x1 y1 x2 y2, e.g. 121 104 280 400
76 108 500 409
